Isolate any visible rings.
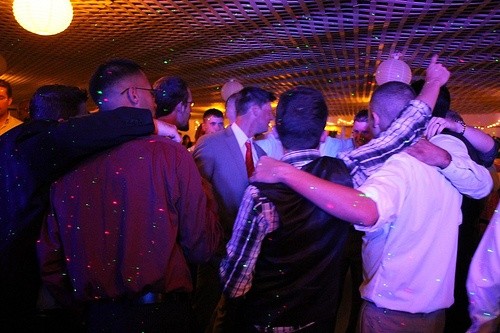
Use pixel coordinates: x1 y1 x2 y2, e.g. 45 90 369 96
436 122 441 127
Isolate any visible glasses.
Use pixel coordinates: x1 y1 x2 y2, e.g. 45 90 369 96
121 87 166 100
184 101 195 107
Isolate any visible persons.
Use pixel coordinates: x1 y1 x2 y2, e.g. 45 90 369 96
151 76 374 159
409 79 500 333
0 84 183 333
206 55 450 333
186 86 276 333
249 80 470 333
466 201 500 333
36 58 225 333
0 80 24 136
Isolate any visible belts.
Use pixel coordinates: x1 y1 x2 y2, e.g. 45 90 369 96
130 293 170 305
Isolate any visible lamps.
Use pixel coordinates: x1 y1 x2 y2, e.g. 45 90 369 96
12 0 73 36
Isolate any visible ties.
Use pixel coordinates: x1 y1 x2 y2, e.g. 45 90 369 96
245 142 254 177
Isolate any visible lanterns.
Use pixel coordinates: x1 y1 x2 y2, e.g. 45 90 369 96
374 59 412 86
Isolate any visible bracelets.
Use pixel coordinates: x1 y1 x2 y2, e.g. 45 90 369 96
460 123 466 135
